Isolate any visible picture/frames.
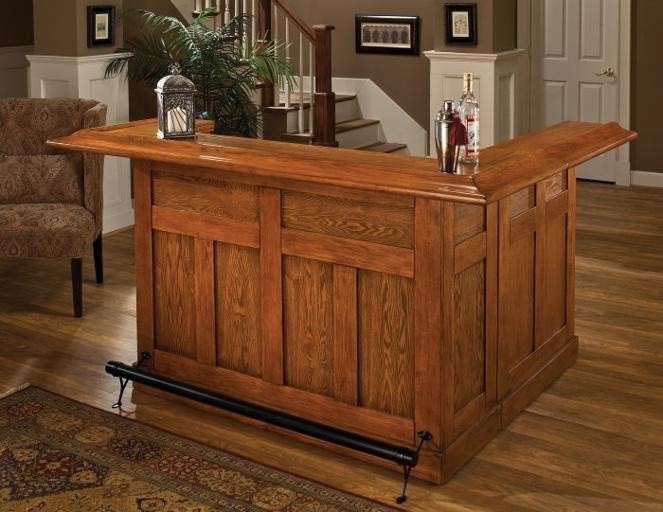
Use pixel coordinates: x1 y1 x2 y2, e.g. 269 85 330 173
444 3 477 45
87 5 116 48
354 14 420 55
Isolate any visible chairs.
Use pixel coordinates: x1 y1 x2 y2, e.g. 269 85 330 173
0 96 108 318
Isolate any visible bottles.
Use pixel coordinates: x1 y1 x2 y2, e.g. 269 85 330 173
433 98 466 175
459 70 484 168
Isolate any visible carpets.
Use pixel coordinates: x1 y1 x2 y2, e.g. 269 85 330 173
1 384 412 512
